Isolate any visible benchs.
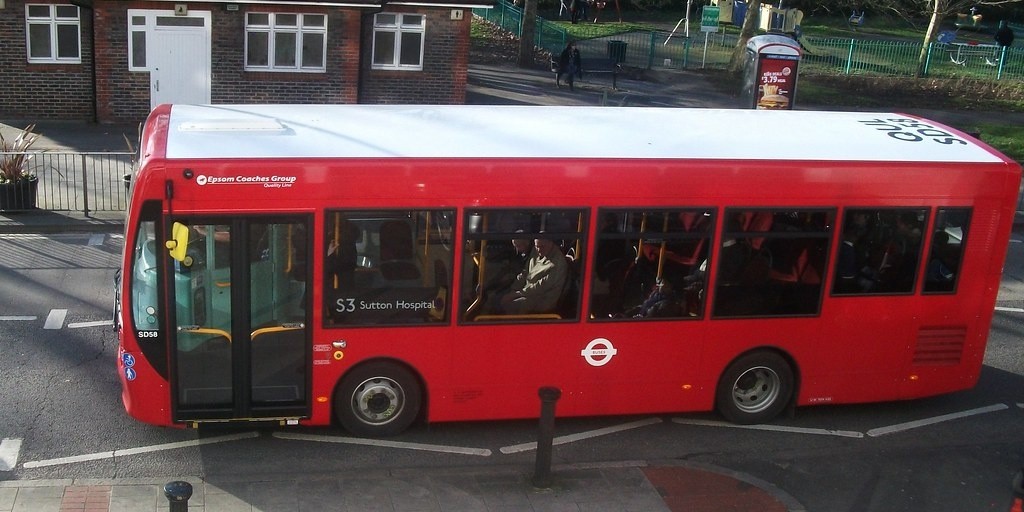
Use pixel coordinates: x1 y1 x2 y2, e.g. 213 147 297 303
549 54 621 91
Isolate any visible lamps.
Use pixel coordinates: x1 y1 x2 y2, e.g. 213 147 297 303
226 4 240 11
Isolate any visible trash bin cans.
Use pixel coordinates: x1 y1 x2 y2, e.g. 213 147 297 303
606 39 629 65
935 31 956 61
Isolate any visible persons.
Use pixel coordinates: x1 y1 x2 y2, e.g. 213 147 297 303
994 20 1014 68
467 211 922 319
193 222 267 269
571 0 581 24
555 41 581 90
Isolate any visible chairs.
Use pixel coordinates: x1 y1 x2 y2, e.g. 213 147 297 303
363 210 921 286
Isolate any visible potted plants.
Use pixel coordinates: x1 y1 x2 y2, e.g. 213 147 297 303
0 122 66 216
122 122 142 191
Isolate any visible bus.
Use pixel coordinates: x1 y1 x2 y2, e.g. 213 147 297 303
111 105 1020 439
